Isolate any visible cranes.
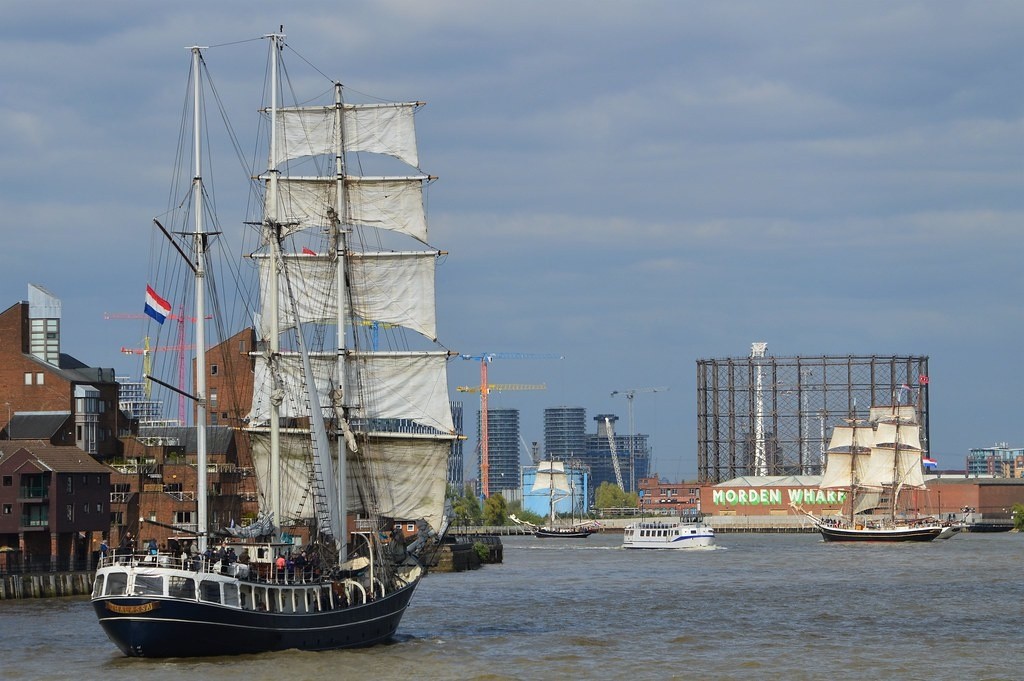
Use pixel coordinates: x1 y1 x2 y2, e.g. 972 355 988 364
610 386 672 493
457 352 570 499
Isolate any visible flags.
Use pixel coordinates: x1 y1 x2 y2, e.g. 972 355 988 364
922 456 938 468
142 284 171 326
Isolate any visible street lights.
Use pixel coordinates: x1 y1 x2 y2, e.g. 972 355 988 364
640 499 644 522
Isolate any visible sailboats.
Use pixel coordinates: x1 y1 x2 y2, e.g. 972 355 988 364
92 25 468 658
508 451 595 538
788 374 971 542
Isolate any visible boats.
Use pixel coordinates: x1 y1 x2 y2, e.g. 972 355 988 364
621 522 717 548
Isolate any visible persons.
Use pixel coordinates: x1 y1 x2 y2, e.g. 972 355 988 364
149 537 159 567
273 551 308 583
99 542 110 559
171 535 201 571
821 516 841 527
203 541 251 576
121 531 135 564
